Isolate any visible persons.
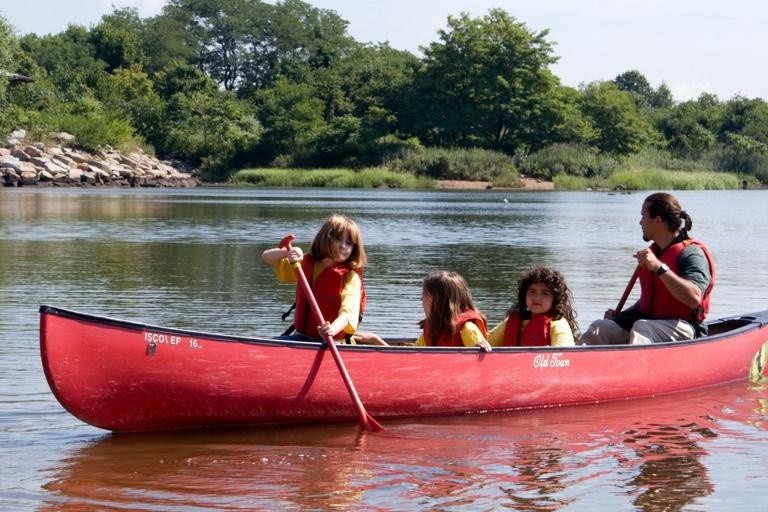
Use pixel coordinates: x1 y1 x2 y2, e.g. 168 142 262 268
356 268 492 355
259 207 367 347
292 423 363 510
484 265 582 346
572 191 716 346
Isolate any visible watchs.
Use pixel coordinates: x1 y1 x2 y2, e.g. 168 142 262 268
655 263 669 277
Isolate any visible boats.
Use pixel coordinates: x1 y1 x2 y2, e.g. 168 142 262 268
39 304 767 433
